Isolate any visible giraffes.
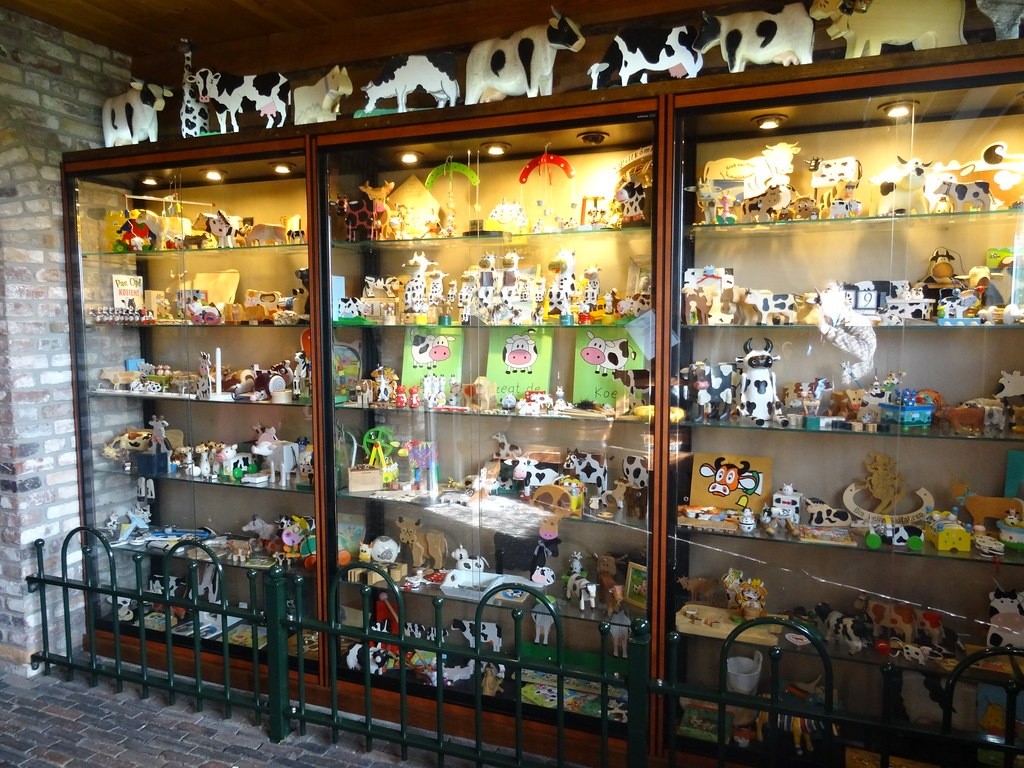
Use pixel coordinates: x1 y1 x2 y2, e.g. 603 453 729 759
176 35 211 140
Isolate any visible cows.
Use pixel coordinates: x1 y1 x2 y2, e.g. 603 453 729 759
808 0 970 61
101 75 174 147
99 142 1024 757
690 0 817 74
463 5 587 106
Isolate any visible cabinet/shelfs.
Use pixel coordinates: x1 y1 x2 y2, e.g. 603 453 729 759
57 37 1024 768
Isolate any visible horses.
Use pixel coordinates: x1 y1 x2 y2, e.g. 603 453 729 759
293 64 354 126
